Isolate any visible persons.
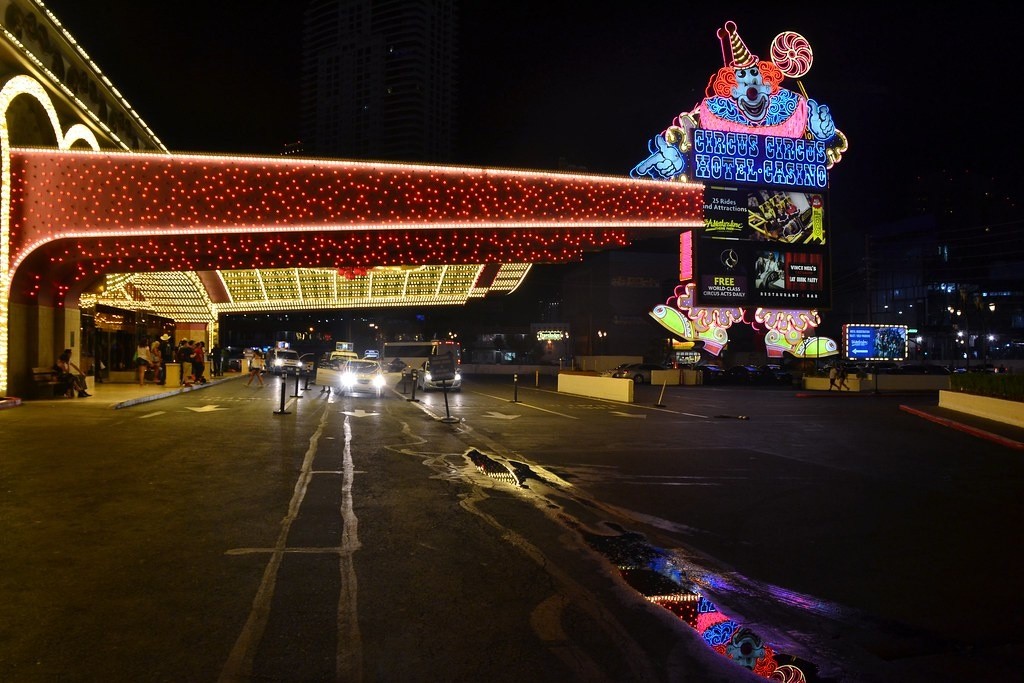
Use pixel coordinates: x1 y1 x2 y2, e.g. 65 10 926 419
242 349 265 388
94 348 104 383
855 362 871 368
321 356 331 392
999 364 1005 373
264 349 271 377
828 362 851 391
209 343 222 377
51 349 92 398
137 334 206 386
391 358 401 372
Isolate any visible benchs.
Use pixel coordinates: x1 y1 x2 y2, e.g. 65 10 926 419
31 367 74 400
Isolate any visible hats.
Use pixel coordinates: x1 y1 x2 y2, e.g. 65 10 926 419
160 334 171 341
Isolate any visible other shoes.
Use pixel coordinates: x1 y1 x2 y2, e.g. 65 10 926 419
78 390 92 397
243 382 250 387
185 383 192 386
257 385 264 388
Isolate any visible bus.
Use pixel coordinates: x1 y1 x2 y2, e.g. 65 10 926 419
381 339 462 382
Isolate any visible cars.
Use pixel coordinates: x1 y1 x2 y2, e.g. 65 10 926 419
316 350 383 399
600 362 663 385
416 361 463 392
666 363 1009 386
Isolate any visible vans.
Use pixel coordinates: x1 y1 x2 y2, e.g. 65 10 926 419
242 348 315 377
